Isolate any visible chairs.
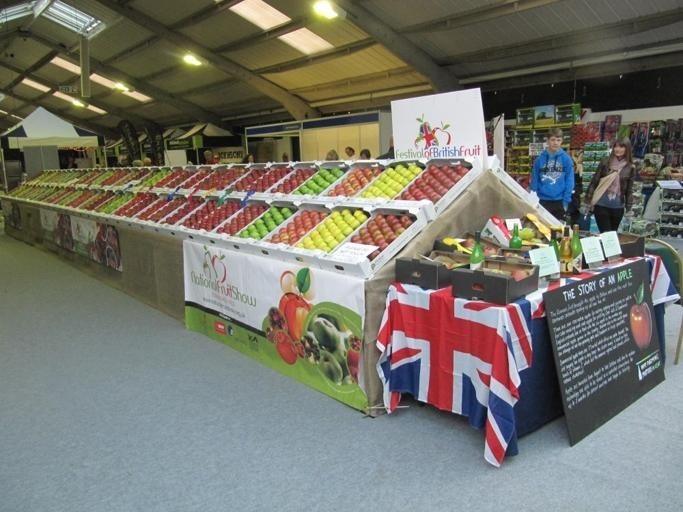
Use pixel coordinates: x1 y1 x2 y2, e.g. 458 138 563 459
645 239 683 362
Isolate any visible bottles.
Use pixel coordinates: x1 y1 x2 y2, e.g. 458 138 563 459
470 231 484 271
549 224 583 278
509 223 522 248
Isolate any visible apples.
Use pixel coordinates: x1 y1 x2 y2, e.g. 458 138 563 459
115 193 328 247
514 268 531 282
413 114 451 149
519 227 536 240
464 238 476 250
395 164 469 203
484 246 496 257
155 165 384 197
629 281 653 349
269 270 360 386
351 213 414 260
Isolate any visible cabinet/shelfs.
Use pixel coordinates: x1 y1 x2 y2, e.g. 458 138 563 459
0 170 548 418
485 123 683 241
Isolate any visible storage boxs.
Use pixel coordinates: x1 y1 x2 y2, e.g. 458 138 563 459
1 154 566 276
393 230 646 305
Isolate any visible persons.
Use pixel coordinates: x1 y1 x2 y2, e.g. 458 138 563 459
566 157 584 229
212 154 219 165
529 127 574 223
203 150 215 165
344 146 358 160
65 155 77 168
324 148 338 161
282 151 288 162
359 149 370 161
241 153 254 163
583 135 635 235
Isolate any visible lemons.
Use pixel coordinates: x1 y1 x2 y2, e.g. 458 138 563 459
296 209 367 253
360 163 422 200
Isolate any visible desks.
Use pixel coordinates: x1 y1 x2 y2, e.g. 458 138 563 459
376 255 680 466
